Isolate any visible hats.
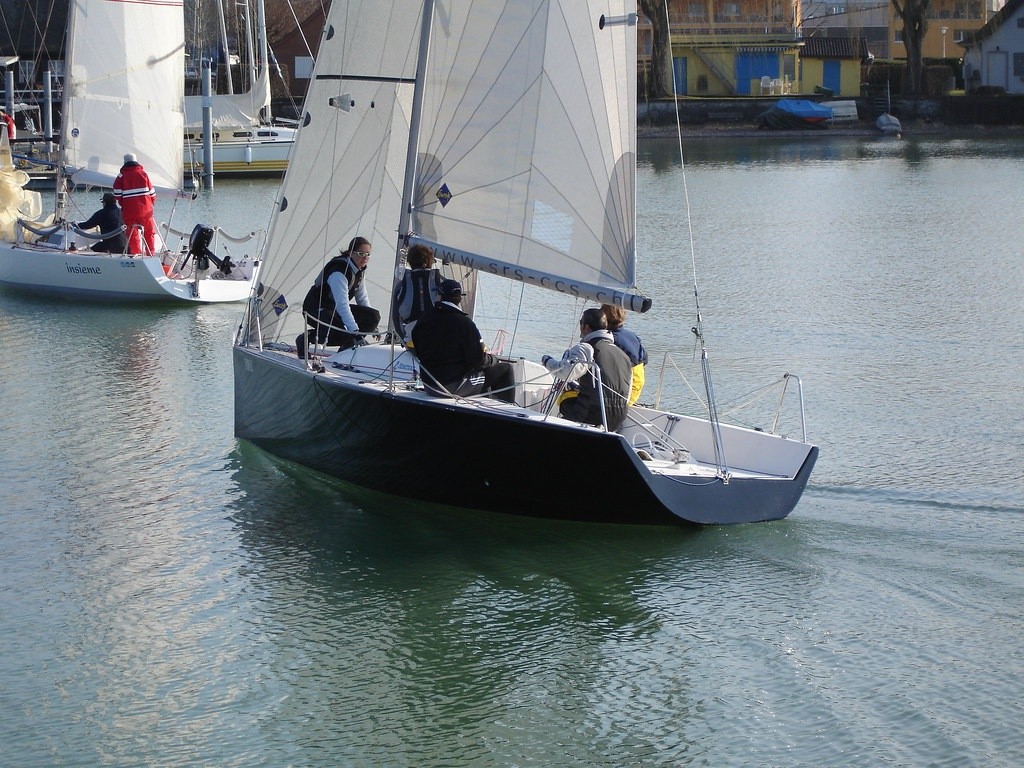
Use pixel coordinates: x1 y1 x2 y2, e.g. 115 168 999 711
100 192 116 203
432 279 462 299
124 153 137 164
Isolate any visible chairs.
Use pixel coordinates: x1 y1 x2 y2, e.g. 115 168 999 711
760 76 784 95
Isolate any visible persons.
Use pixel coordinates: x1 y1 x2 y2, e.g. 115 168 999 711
295 236 381 360
391 244 515 405
541 304 649 434
72 193 126 255
114 153 157 256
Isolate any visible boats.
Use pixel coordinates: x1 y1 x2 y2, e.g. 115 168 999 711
817 98 859 127
755 98 834 130
874 112 902 131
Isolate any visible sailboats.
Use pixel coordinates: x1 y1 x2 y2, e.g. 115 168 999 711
181 0 331 178
231 0 822 531
0 0 261 303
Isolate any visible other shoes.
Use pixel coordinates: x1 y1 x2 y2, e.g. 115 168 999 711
296 334 311 359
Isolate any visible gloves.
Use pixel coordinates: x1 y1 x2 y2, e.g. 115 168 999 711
542 355 552 366
352 331 370 348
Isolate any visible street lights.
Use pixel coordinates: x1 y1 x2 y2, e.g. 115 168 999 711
938 26 950 59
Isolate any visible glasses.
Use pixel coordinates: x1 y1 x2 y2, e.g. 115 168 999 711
579 319 587 325
352 251 372 258
